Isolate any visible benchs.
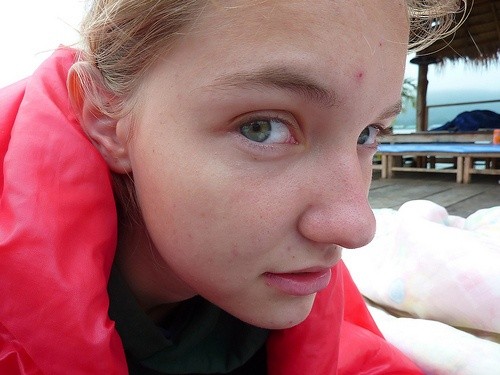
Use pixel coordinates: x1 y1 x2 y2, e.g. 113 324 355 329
372 144 500 185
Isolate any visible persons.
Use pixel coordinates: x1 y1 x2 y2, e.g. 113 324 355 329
0 0 435 375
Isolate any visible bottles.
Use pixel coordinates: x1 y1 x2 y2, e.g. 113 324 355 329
494 130 500 144
